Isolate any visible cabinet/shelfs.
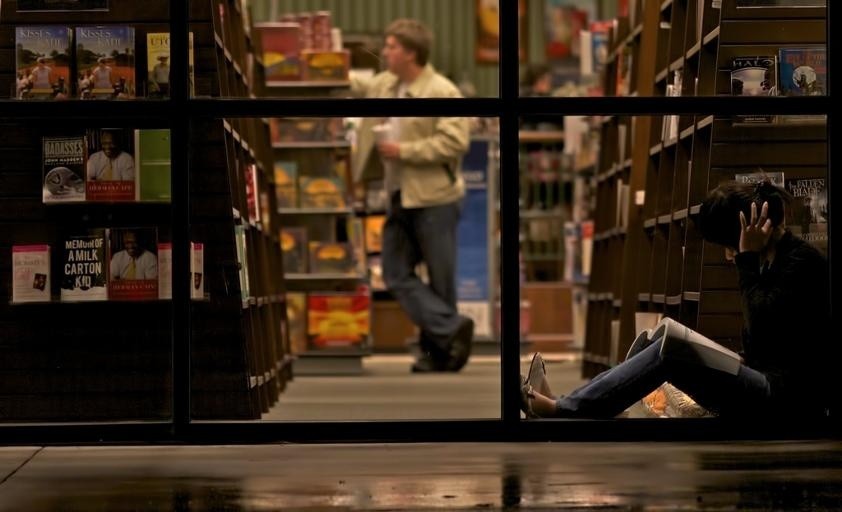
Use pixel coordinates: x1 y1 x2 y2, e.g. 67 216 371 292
255 5 420 368
487 70 585 355
1 0 292 421
582 0 840 374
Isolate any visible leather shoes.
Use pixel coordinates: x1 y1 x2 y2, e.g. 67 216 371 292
527 351 549 396
520 372 536 420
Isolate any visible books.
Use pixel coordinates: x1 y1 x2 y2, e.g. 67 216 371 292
559 21 633 369
659 69 681 141
728 46 828 256
230 161 273 302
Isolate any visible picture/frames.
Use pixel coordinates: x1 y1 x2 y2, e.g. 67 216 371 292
474 0 530 65
539 2 591 64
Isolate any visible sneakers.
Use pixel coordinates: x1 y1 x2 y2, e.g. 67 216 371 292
411 315 474 373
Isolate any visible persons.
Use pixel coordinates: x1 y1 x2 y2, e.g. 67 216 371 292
519 179 840 417
109 231 159 281
347 19 473 373
87 131 134 181
152 52 170 99
17 56 135 102
